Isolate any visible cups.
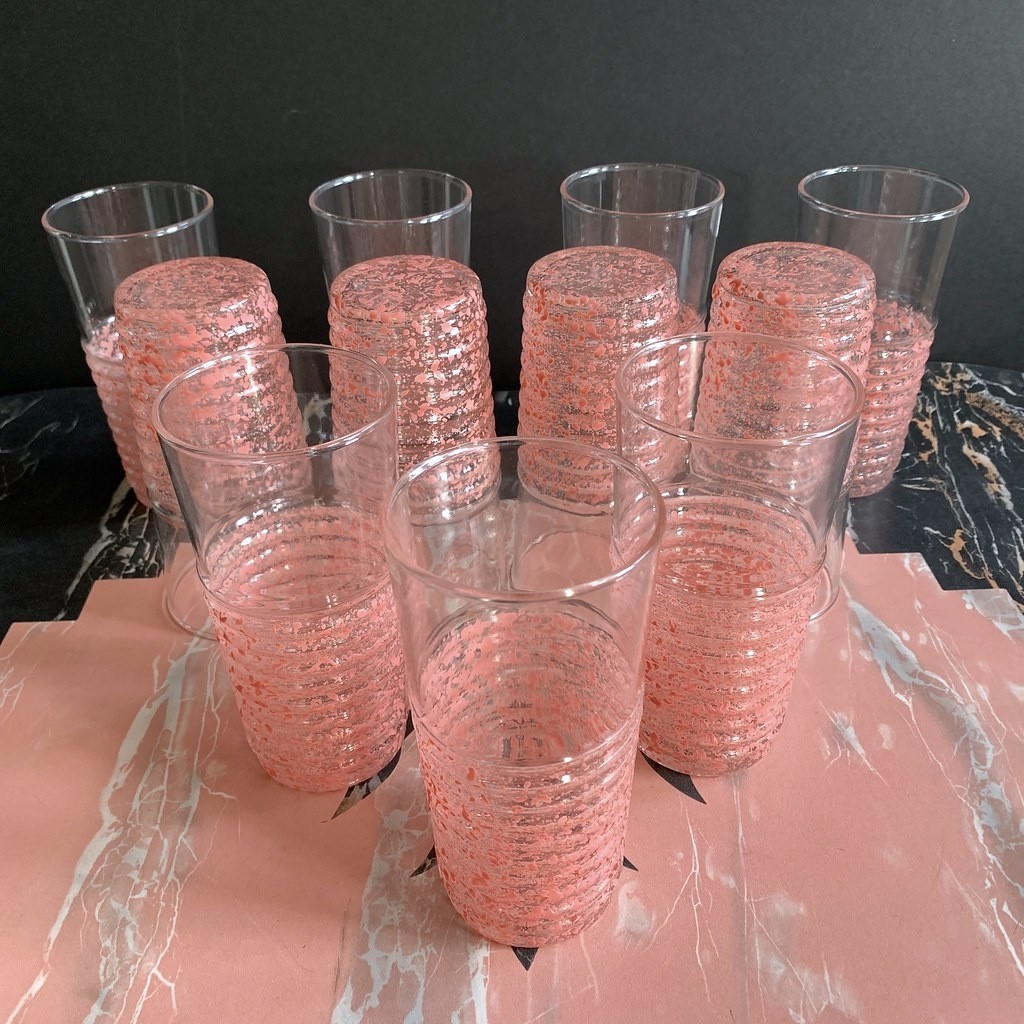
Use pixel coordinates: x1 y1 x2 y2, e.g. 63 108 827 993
688 239 877 617
381 435 668 950
311 165 472 305
560 162 725 433
38 180 221 509
519 250 681 530
151 342 410 793
616 333 864 776
113 257 315 641
324 254 511 610
796 163 971 496
